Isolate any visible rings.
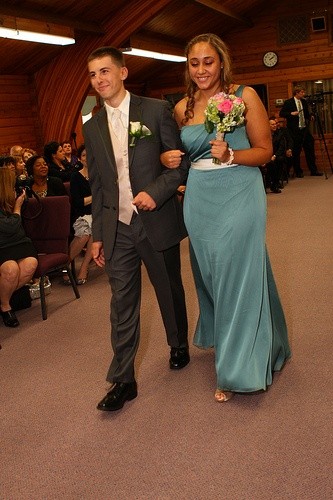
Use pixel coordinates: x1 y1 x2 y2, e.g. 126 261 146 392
182 190 184 192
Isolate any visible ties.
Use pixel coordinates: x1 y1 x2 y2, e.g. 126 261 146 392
298 101 304 127
113 110 135 226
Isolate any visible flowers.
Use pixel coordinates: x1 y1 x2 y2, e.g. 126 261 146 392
204 92 246 164
129 112 152 147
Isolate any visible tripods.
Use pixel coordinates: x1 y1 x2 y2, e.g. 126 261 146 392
288 111 333 180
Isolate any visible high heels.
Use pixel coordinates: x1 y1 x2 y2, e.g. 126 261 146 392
77 271 88 285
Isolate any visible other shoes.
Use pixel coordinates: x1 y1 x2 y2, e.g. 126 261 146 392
215 390 233 404
280 184 284 188
297 174 304 177
2 310 19 327
310 172 323 176
64 278 71 285
270 188 281 193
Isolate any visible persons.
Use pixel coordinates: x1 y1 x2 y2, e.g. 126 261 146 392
156 34 290 403
0 85 322 326
82 47 188 411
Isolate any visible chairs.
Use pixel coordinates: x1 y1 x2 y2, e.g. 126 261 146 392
21 196 80 320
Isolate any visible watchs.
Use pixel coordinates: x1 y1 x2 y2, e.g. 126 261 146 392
274 155 276 158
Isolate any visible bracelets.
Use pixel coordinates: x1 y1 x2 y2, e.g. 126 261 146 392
226 147 235 166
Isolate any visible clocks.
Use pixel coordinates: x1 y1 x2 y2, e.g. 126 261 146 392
263 51 278 68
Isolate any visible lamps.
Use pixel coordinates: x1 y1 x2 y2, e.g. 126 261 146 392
0 13 75 46
119 39 188 63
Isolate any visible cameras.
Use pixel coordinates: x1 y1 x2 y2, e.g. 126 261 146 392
15 176 34 198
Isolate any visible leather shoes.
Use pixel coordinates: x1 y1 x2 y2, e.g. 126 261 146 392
97 380 137 412
170 346 190 369
